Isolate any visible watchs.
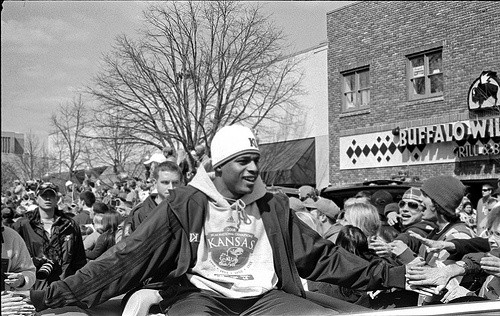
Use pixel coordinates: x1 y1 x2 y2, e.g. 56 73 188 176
456 261 468 269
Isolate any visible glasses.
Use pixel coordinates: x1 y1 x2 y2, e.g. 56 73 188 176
398 200 421 210
482 189 488 192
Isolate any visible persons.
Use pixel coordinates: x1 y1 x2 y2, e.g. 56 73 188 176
22 125 442 316
289 176 500 304
1 146 208 316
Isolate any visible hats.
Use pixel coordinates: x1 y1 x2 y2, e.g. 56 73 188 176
370 190 393 216
303 198 341 220
420 175 466 216
1 207 14 219
35 182 60 198
289 197 306 212
402 187 422 201
210 124 261 169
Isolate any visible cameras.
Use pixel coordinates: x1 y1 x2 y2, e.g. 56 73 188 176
36 257 62 281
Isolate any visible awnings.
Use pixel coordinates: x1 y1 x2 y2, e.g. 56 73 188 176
258 138 315 186
